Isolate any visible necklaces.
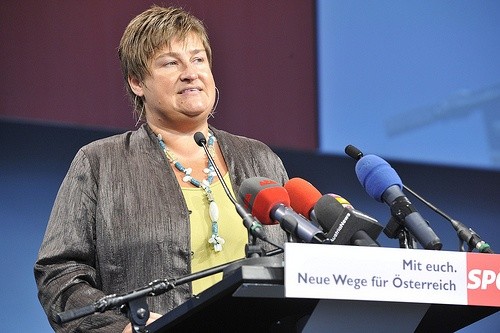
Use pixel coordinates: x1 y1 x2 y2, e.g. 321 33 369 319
155 132 225 251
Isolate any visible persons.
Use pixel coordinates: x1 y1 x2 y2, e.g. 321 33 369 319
33 2 289 333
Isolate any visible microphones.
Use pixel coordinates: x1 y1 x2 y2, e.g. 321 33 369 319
194 132 494 257
